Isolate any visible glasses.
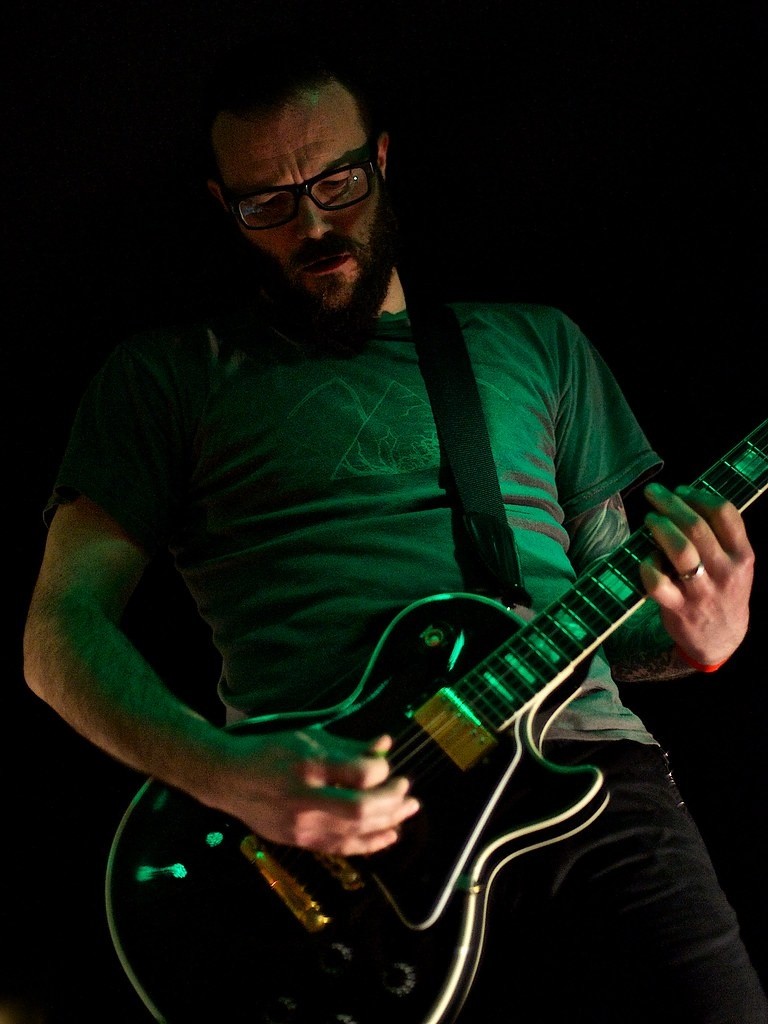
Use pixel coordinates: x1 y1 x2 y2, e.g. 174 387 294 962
218 143 380 231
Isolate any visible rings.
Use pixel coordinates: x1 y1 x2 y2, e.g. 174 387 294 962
675 563 705 582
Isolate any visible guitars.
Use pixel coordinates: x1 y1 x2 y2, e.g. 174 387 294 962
98 411 767 1024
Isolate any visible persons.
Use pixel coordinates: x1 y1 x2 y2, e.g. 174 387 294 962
23 59 768 1024
